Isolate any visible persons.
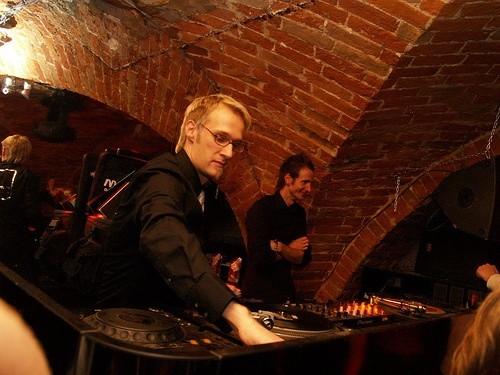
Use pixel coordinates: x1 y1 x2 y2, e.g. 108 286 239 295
244 152 314 300
90 93 285 375
450 263 500 375
0 299 51 375
46 179 75 210
0 133 54 311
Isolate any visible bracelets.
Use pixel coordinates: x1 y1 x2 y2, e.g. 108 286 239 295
273 239 278 252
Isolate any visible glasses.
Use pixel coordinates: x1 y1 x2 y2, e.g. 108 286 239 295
199 123 244 153
